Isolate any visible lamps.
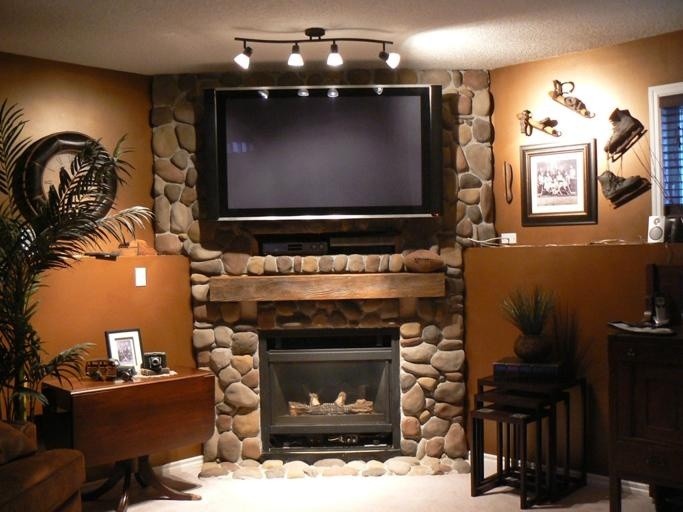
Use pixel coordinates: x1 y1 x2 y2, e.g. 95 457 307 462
297 90 309 97
288 42 303 66
327 89 339 97
259 90 269 98
327 41 343 68
373 88 383 95
233 40 252 70
378 43 400 70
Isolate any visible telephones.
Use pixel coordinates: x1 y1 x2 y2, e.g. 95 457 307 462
653 290 669 329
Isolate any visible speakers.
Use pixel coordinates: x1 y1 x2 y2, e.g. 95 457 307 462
646 215 665 244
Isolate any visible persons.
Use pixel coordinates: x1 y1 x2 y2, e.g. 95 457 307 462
538 167 573 197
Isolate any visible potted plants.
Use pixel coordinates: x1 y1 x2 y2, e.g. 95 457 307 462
502 285 552 360
0 99 156 421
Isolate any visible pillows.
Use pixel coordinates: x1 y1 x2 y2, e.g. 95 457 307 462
0 421 38 466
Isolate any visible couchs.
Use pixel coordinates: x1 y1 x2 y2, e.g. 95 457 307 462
0 420 85 512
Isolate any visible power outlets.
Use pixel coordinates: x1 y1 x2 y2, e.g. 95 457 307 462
135 267 146 287
501 233 517 244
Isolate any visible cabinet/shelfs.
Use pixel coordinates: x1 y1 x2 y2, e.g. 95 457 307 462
610 264 683 512
41 366 215 512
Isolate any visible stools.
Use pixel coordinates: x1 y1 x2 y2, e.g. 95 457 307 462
474 387 568 497
472 408 549 512
476 375 588 495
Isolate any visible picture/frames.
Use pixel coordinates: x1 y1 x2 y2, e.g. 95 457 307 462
520 138 598 227
105 328 144 377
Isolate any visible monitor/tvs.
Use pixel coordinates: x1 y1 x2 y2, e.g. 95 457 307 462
211 84 442 222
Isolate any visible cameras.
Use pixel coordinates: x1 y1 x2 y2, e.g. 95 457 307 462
143 353 167 371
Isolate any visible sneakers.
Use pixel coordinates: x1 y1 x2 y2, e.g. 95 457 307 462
600 107 640 153
598 171 640 200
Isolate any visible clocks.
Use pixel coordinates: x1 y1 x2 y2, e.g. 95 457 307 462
17 131 117 229
